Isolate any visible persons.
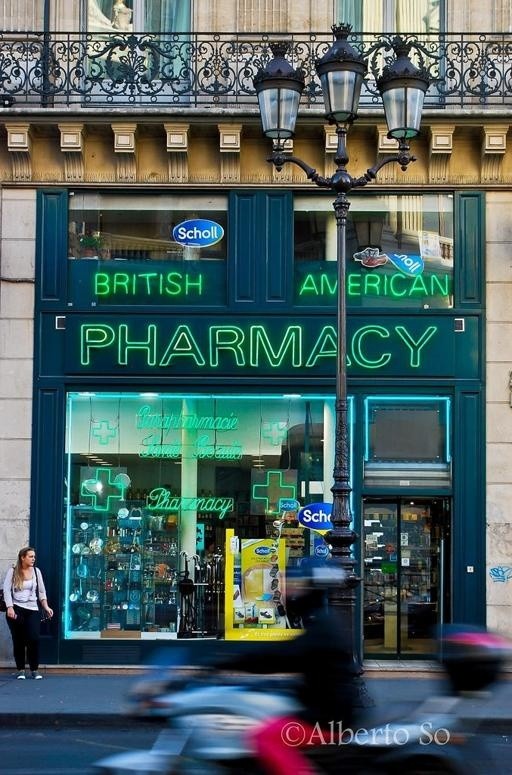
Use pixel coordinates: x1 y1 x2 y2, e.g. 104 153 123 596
3 546 54 680
165 558 376 773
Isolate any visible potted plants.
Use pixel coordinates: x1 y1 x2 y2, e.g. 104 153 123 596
77 234 102 258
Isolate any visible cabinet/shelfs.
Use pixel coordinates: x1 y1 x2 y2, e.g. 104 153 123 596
68 500 266 632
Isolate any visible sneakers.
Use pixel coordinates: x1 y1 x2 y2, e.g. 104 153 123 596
17 671 25 680
32 671 43 679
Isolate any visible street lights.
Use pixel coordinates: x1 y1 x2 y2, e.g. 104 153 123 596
252 23 433 676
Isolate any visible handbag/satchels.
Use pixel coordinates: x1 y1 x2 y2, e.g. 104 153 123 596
0 566 16 612
38 601 51 625
